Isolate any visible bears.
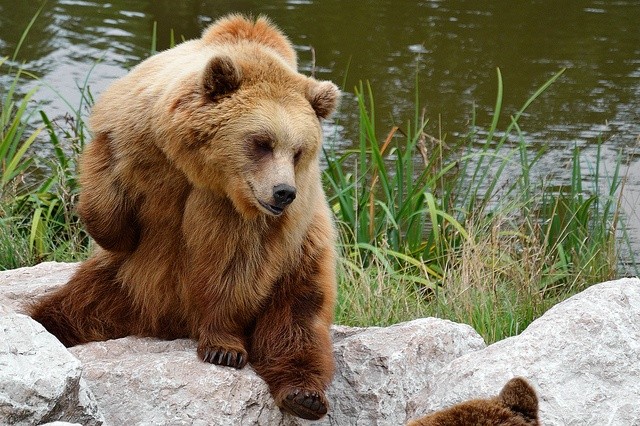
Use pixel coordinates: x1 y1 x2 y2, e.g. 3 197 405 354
403 377 541 426
18 10 342 422
